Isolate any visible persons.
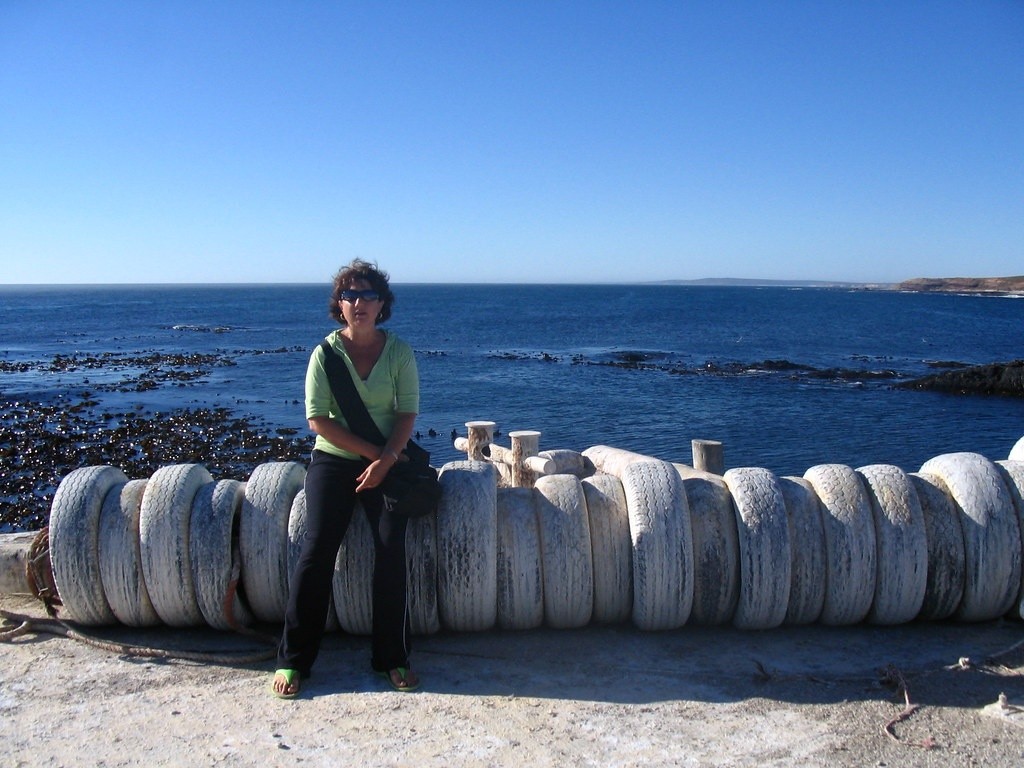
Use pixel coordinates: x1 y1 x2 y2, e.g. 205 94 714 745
270 261 420 699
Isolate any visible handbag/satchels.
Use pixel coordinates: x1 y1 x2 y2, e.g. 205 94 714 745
359 438 444 518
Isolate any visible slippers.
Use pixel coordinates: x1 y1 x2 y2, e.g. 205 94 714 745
372 664 422 691
271 667 303 699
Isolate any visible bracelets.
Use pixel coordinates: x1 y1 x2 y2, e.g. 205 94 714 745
389 447 398 460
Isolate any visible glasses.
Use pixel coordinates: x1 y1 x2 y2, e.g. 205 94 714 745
339 289 381 302
